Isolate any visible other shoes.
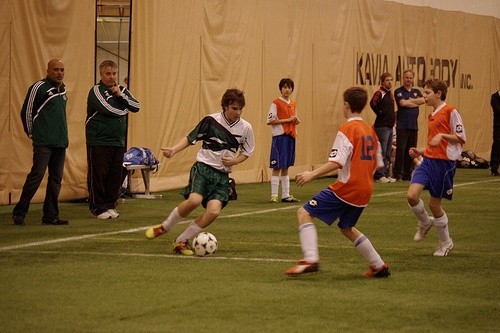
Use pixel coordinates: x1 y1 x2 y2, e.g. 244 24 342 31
12 209 25 225
366 263 391 278
270 197 278 202
433 240 453 256
376 177 396 183
285 258 320 277
145 224 168 239
280 194 301 202
491 172 500 176
172 239 194 255
96 208 119 219
414 217 434 241
42 216 68 225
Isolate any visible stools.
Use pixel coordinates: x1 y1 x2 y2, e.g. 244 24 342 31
126 163 161 200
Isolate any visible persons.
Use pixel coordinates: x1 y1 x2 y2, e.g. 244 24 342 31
144 90 256 256
265 78 300 204
408 80 466 256
370 70 500 182
86 60 140 220
285 87 391 278
13 59 70 225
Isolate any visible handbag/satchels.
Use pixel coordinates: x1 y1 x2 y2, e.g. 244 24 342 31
123 146 160 174
228 178 237 200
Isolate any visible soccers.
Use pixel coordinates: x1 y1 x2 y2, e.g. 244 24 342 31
191 231 218 257
